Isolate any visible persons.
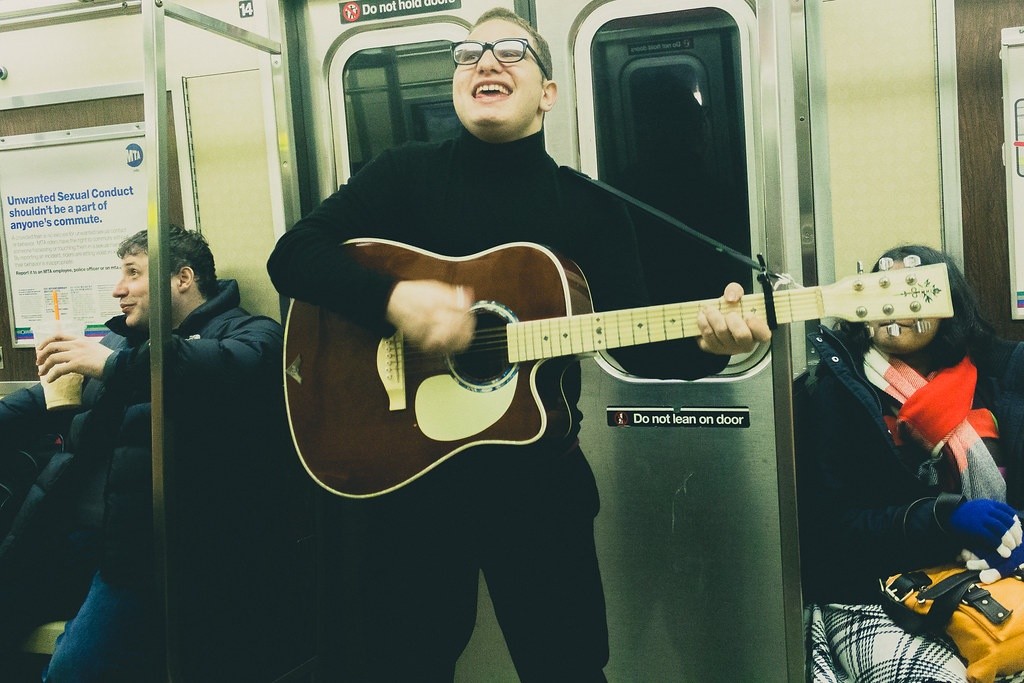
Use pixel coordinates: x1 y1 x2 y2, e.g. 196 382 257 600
784 242 1024 683
1 223 280 683
268 6 773 683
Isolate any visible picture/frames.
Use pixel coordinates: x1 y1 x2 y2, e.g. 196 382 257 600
0 122 146 349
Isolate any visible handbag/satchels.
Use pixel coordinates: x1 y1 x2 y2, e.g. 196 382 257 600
881 564 1023 681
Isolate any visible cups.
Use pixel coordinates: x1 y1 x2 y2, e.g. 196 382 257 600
31 319 85 411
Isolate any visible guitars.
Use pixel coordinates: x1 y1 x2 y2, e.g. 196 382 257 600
283 237 954 500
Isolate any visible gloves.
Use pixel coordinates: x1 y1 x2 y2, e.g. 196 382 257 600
963 530 1024 582
950 499 1023 558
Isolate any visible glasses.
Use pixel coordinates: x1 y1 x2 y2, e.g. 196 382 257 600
450 37 551 84
